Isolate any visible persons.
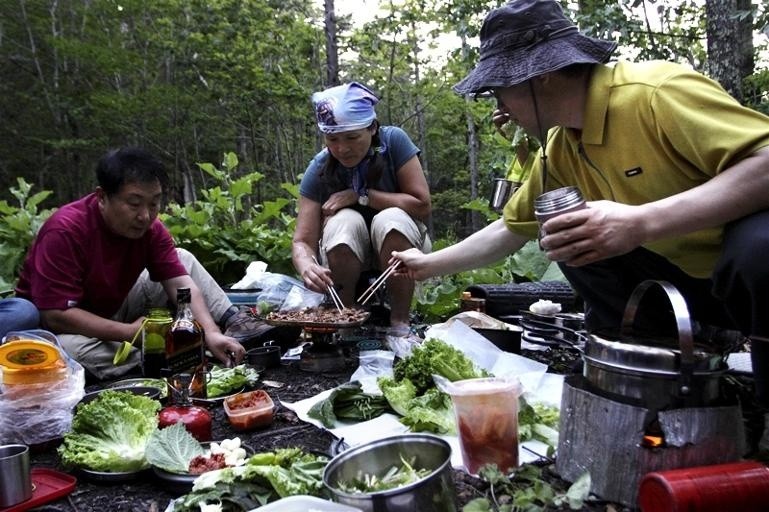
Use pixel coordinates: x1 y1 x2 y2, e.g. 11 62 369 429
292 83 432 342
493 110 552 283
0 298 39 336
15 148 302 380
388 2 768 335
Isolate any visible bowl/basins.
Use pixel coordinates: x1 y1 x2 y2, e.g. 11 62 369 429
322 435 460 512
248 345 280 367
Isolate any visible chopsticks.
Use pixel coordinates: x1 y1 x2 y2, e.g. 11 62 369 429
356 259 402 306
310 255 346 316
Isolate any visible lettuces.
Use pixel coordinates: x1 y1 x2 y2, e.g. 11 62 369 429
377 339 560 441
57 390 160 472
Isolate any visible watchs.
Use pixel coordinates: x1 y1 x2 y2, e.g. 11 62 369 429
359 189 370 206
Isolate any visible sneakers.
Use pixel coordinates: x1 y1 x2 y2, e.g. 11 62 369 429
224 310 302 351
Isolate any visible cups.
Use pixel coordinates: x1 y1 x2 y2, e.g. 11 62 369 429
447 378 518 478
0 444 32 510
534 186 588 263
113 307 174 378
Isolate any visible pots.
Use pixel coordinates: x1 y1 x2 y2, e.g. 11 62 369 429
520 279 730 436
489 178 524 210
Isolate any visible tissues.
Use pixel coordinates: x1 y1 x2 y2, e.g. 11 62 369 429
224 260 268 305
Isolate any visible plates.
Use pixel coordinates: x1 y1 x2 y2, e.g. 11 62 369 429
73 378 255 490
260 309 371 329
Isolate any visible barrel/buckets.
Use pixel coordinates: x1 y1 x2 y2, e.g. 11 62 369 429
572 279 729 408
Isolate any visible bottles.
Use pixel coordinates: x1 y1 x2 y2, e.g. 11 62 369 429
637 460 768 510
166 287 208 408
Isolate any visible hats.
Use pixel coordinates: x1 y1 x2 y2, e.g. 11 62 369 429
451 0 618 95
